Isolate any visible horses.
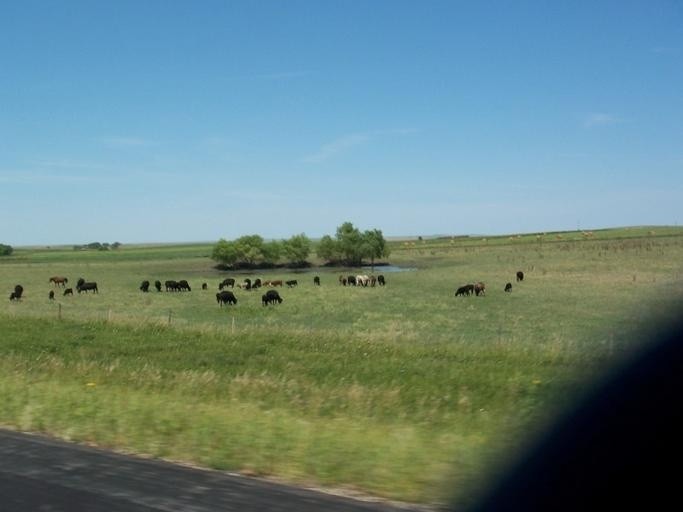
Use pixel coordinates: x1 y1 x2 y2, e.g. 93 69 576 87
49 277 67 288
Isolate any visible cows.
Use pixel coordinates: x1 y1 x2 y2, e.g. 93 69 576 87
216 290 237 308
8 284 23 299
337 274 386 287
63 288 73 296
217 277 299 290
49 290 54 300
141 280 191 292
261 290 283 307
453 282 486 297
504 283 513 292
313 276 321 286
516 272 523 281
76 277 98 294
